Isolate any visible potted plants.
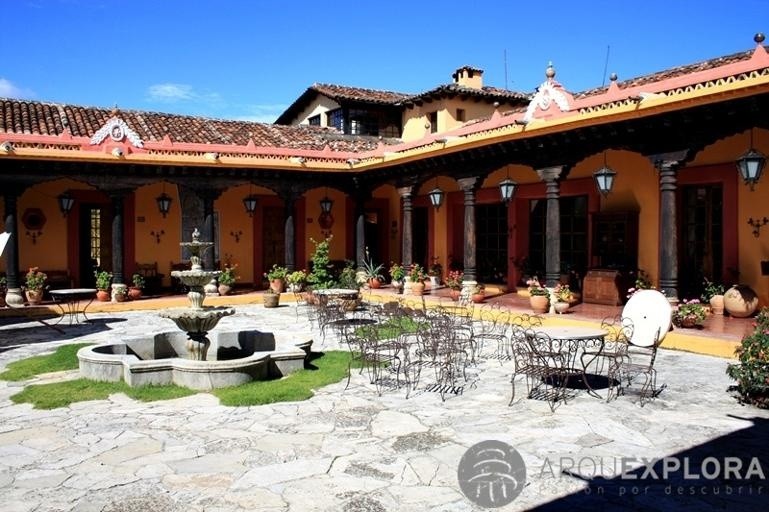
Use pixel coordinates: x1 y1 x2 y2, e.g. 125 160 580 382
286 270 307 292
94 270 147 302
360 257 387 289
701 277 725 315
0 277 9 307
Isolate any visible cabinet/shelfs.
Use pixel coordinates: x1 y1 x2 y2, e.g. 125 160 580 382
588 210 639 304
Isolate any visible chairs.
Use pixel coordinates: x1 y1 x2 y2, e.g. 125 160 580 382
292 277 667 412
138 262 164 293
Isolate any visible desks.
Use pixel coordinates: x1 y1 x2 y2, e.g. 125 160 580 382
49 289 99 328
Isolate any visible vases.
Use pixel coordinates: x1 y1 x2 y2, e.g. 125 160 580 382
218 284 232 296
25 290 43 305
270 278 284 293
554 301 569 312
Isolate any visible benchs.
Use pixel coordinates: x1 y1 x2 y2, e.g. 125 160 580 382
1 270 68 300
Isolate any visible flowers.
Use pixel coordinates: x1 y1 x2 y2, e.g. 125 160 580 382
20 267 50 291
217 262 241 285
389 260 572 304
625 268 707 328
726 304 769 409
262 263 290 282
